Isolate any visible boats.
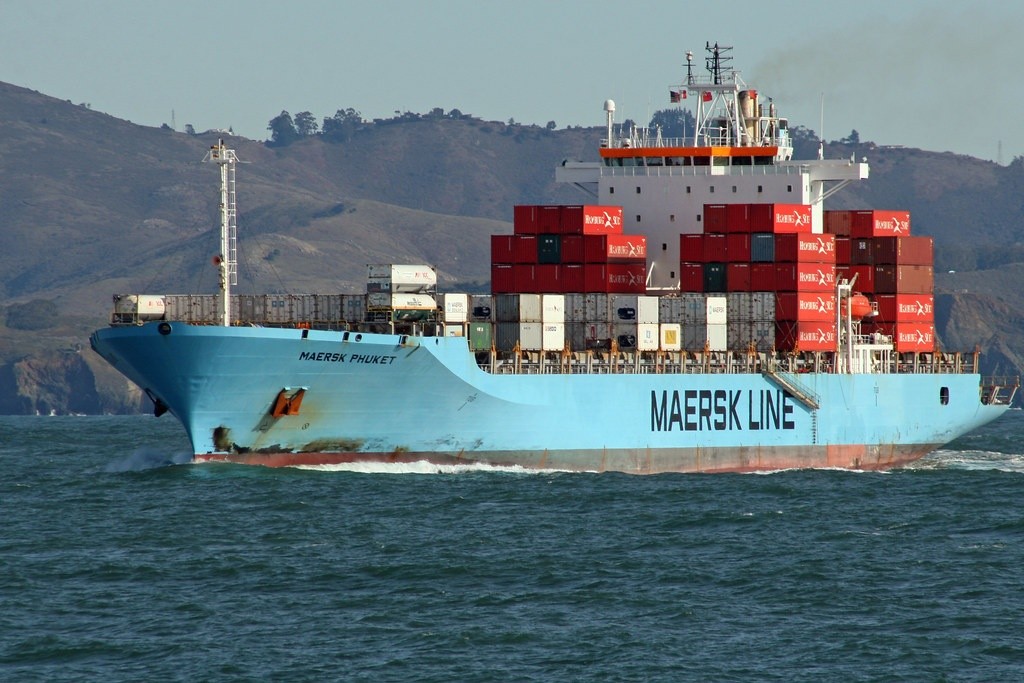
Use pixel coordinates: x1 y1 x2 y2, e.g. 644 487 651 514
84 42 1023 476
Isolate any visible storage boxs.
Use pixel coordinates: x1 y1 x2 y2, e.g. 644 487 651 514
164 295 367 322
491 206 648 293
824 210 934 354
565 292 777 352
681 203 836 352
435 294 563 351
